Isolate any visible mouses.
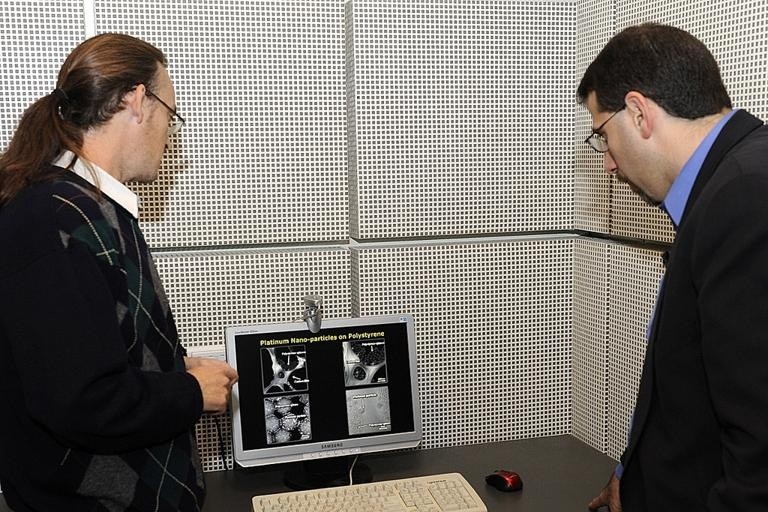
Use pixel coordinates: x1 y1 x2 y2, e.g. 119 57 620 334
485 469 523 492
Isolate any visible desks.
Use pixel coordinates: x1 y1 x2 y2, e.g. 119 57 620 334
200 435 620 512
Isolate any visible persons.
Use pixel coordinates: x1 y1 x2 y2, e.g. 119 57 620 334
1 33 240 511
577 23 768 511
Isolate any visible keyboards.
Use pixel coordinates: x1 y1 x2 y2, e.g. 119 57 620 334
251 471 488 512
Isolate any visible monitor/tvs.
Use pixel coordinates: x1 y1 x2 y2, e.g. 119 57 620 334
223 314 422 490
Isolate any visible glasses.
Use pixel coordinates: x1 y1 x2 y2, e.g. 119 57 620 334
584 102 635 154
124 84 187 138
